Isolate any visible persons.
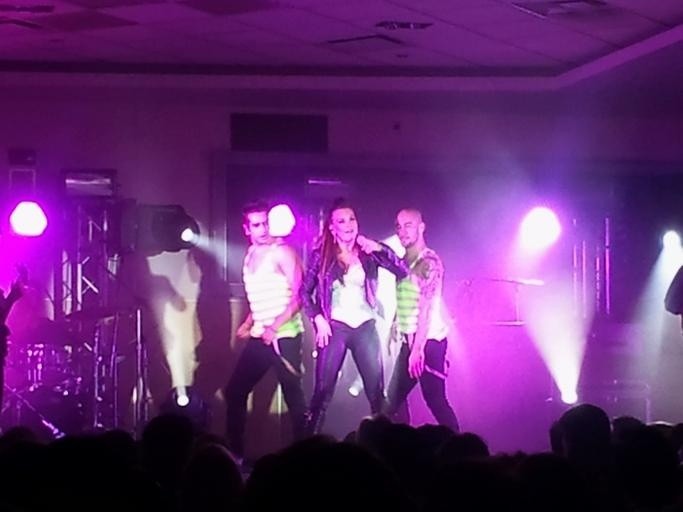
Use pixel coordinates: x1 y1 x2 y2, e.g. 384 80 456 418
299 197 406 439
221 199 307 456
0 267 28 414
1 401 683 511
384 204 459 431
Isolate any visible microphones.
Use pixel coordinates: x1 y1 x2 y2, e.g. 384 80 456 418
356 234 383 265
